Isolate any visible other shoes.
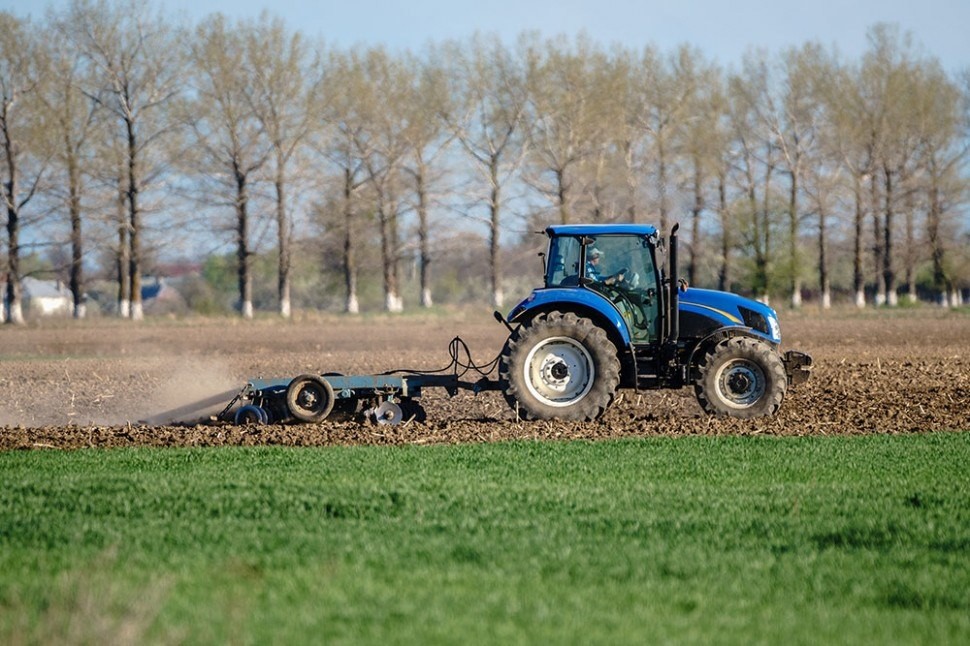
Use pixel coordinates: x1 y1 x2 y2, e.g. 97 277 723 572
634 329 647 339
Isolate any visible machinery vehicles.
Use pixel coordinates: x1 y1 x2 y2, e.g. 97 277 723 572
479 223 812 425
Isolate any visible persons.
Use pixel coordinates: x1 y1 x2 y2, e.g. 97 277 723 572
586 248 625 298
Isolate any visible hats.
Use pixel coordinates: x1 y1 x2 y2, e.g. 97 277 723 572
586 248 604 257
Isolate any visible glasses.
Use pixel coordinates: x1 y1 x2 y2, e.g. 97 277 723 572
592 255 600 260
